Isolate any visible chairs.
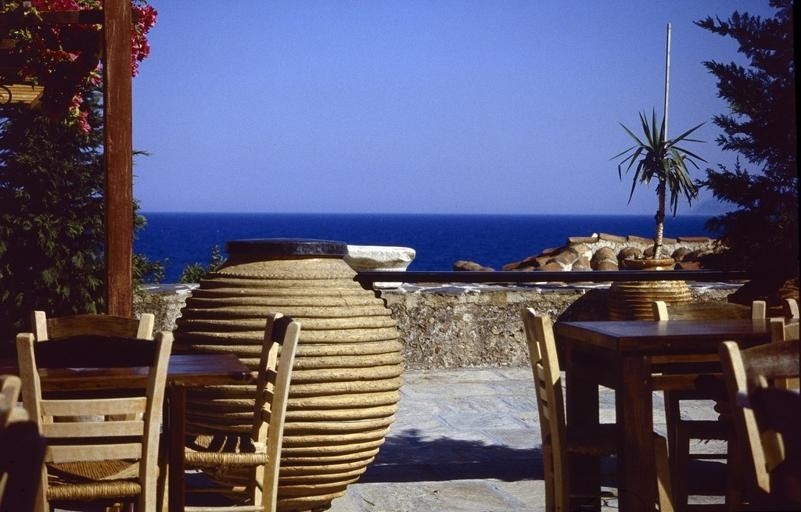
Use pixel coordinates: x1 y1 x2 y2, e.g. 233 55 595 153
160 312 297 511
34 310 155 340
521 308 672 511
652 300 800 511
16 332 173 511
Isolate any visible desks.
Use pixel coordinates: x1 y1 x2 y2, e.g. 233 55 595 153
2 337 249 510
554 317 800 511
